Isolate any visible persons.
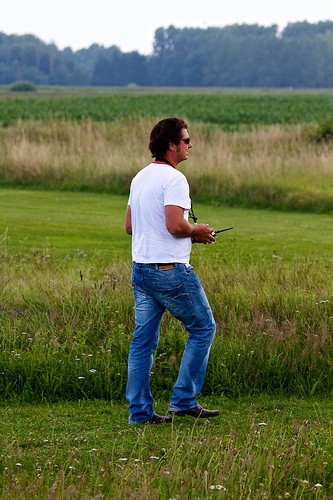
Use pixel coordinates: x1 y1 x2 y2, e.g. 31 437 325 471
125 118 221 429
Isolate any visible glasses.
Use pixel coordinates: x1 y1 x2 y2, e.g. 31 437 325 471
180 138 189 144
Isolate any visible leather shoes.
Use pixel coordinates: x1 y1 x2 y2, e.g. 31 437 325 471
141 405 219 424
